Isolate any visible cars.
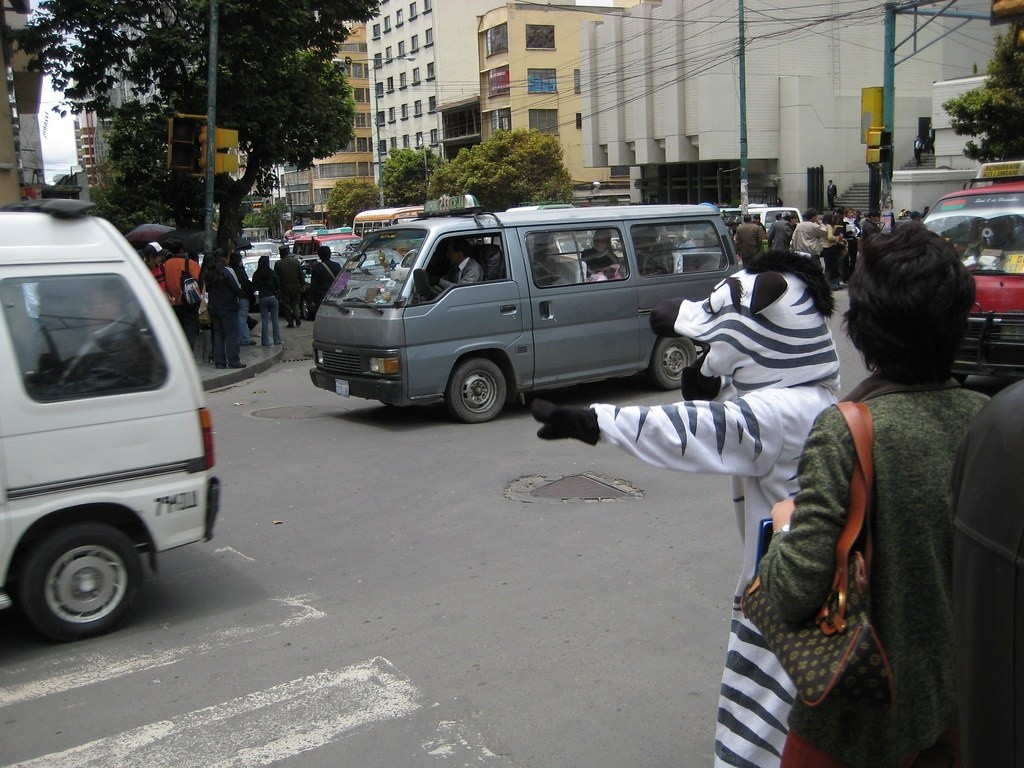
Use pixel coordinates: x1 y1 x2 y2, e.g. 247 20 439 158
240 223 419 284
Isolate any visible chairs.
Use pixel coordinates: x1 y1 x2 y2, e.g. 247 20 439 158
556 256 587 285
471 244 504 280
671 253 683 274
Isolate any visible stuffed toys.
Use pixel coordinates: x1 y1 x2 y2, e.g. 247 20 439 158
532 244 842 767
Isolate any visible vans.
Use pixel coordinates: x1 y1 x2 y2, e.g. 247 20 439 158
351 205 426 238
716 206 741 233
308 194 741 426
0 198 223 646
747 206 805 234
918 159 1024 388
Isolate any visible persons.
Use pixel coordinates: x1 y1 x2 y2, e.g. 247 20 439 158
578 228 622 283
745 222 1023 768
914 123 935 165
33 231 342 412
430 236 485 302
635 227 674 274
725 179 926 289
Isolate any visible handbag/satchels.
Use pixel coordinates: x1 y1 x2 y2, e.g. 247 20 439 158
740 401 897 732
196 280 212 327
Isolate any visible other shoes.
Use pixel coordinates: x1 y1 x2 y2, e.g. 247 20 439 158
286 316 301 328
244 341 256 345
216 362 246 369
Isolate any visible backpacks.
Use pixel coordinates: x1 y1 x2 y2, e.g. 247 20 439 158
179 256 202 307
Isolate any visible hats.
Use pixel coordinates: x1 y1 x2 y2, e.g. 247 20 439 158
143 241 169 257
278 245 289 251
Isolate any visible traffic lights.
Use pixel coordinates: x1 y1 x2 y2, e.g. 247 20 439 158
196 123 241 176
165 116 197 170
864 131 892 168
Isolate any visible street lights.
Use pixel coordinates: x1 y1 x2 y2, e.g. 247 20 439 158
347 55 417 208
